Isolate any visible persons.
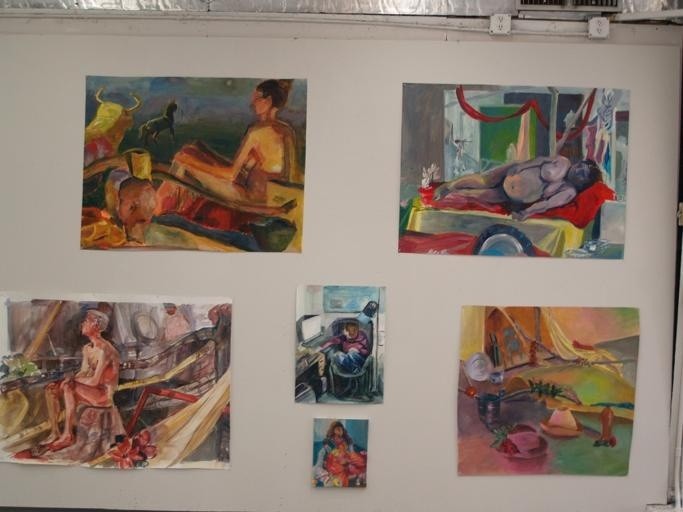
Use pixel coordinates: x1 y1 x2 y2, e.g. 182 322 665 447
154 80 300 213
313 439 332 486
431 153 601 223
314 322 370 373
37 308 118 451
326 422 365 486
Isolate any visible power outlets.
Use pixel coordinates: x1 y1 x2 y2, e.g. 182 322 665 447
588 15 611 39
489 13 511 37
